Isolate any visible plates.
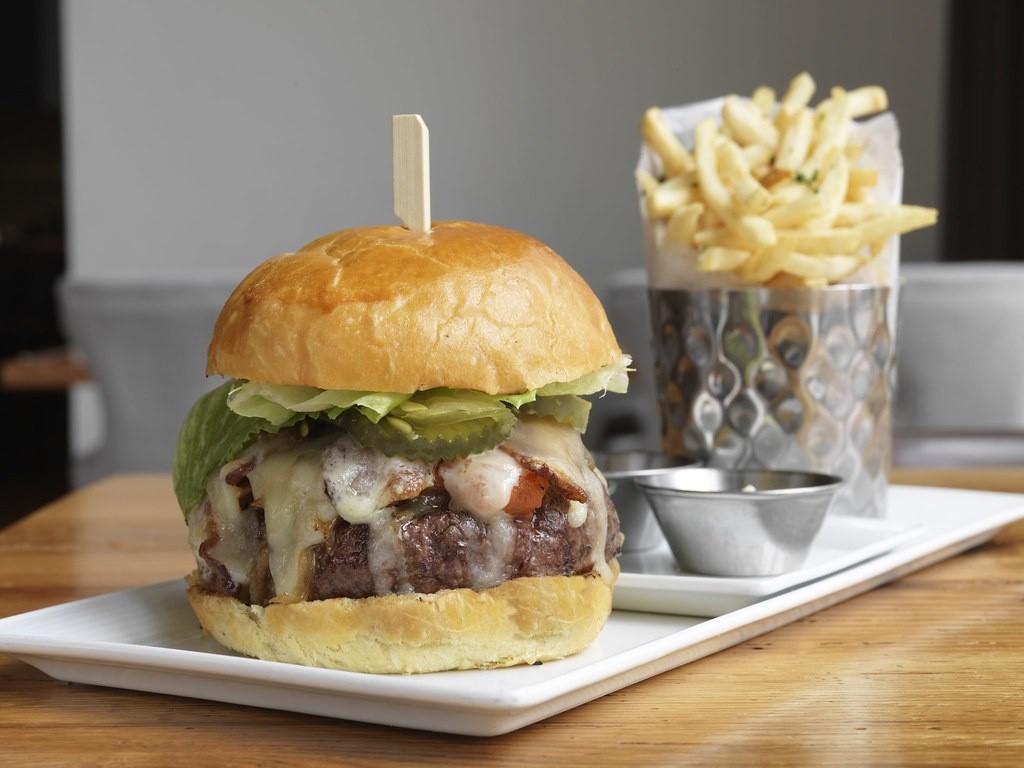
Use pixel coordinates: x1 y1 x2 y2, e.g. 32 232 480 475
0 483 1024 737
615 514 909 622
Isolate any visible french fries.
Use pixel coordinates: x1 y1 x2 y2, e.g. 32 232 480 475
634 72 937 288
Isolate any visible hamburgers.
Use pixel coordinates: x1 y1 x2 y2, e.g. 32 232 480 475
172 218 638 677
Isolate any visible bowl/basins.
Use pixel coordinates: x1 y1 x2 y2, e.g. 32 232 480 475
636 468 845 580
590 448 705 555
604 261 1024 462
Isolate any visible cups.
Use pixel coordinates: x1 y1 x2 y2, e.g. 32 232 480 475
647 284 897 521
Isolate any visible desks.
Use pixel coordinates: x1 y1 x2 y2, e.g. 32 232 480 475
0 461 1024 768
